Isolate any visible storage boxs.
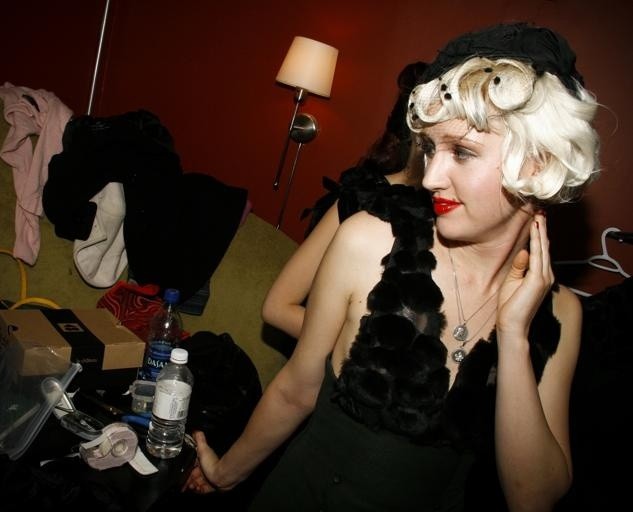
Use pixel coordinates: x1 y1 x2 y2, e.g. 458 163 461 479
2 308 146 377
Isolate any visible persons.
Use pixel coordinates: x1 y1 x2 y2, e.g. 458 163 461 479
261 61 429 338
181 23 582 512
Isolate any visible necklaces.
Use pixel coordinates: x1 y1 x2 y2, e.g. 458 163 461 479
448 242 500 341
452 273 498 363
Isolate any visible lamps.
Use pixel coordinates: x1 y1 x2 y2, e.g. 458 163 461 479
271 36 339 192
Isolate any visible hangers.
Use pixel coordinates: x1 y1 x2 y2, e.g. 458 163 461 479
554 229 630 298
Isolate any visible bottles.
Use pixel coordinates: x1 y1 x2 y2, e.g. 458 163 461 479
146 348 195 460
142 288 182 381
